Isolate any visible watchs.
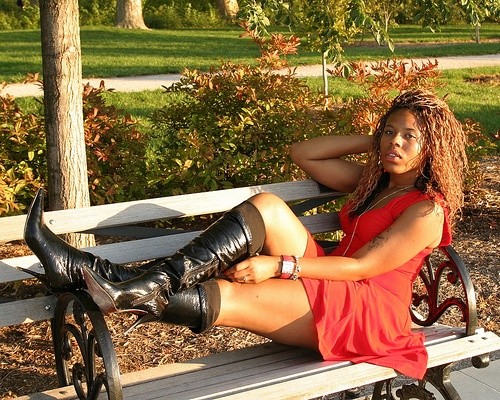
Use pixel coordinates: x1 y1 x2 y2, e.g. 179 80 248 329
280 254 294 279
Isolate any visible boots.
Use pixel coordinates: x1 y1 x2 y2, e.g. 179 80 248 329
83 205 253 337
18 187 210 334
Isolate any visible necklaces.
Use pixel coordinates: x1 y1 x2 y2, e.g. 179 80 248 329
340 183 415 257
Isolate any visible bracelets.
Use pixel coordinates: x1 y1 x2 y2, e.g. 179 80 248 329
291 255 299 281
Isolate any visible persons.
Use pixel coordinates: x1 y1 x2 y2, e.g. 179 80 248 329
23 88 469 379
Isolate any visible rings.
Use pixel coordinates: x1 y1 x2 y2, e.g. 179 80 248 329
242 277 247 282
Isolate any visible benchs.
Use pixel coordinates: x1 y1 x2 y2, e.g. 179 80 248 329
0 177 500 400
